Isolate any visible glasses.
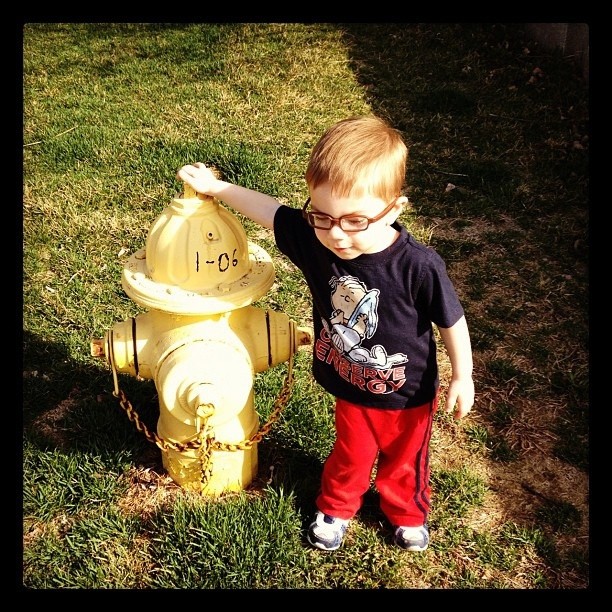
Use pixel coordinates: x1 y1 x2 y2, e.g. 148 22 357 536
302 193 406 232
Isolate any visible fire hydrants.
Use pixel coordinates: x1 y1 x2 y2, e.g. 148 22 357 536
92 172 311 496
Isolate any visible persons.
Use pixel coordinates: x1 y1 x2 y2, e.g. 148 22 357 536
175 116 476 551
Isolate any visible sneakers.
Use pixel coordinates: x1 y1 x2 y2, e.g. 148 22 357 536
308 511 352 551
393 523 430 551
